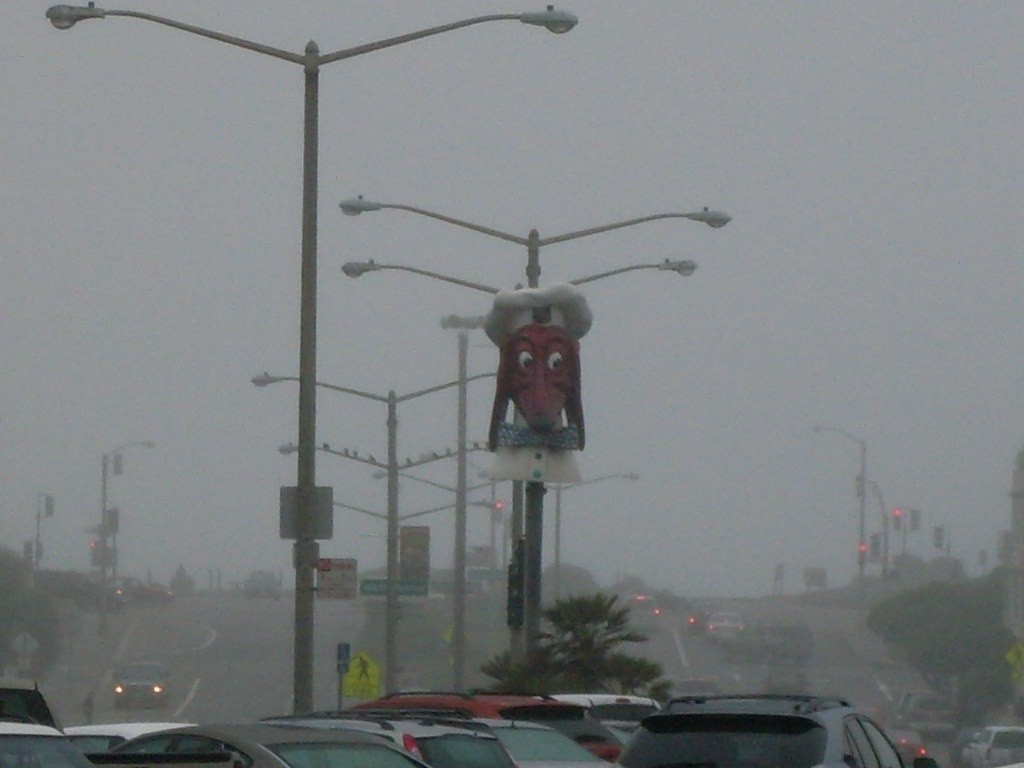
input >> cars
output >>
[113,660,168,710]
[705,612,746,642]
[626,594,660,629]
[0,686,1023,768]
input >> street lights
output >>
[342,259,699,654]
[270,440,644,696]
[252,365,501,697]
[333,189,731,673]
[45,0,580,717]
[812,425,868,632]
[99,437,154,588]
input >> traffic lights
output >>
[493,500,504,523]
[857,544,868,566]
[506,564,524,627]
[893,507,902,531]
